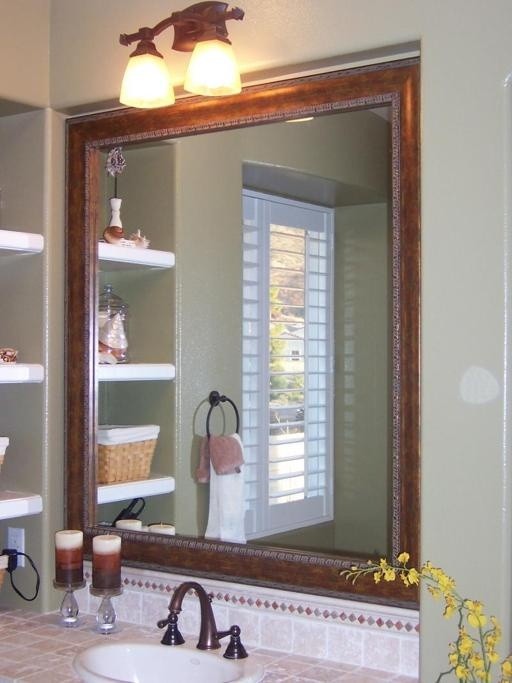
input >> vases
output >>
[109,198,124,228]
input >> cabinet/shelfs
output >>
[95,243,178,508]
[0,230,46,523]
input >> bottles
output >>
[98,283,131,364]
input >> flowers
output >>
[338,555,511,683]
[103,146,128,199]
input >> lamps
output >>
[117,1,248,111]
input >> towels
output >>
[192,429,252,543]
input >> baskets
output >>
[97,425,160,485]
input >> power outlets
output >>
[8,526,26,567]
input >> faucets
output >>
[167,580,220,650]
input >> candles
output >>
[54,531,124,590]
[114,519,175,536]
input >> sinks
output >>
[74,643,241,683]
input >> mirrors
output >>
[58,51,429,614]
